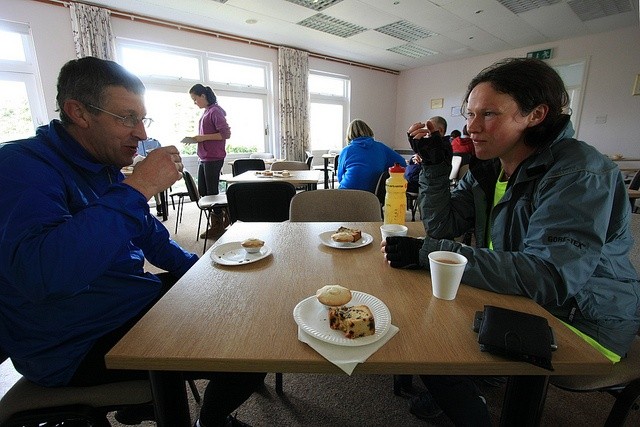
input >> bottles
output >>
[384,163,408,225]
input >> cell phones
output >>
[473,309,559,351]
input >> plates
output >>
[293,291,390,347]
[209,242,272,266]
[317,230,373,249]
[257,175,273,178]
[274,174,294,177]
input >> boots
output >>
[200,212,224,238]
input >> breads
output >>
[334,226,363,242]
[328,305,377,339]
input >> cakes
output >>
[331,233,354,246]
[315,284,352,307]
[242,237,265,253]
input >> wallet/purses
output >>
[477,305,555,372]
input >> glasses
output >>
[87,102,152,128]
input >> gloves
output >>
[408,130,444,164]
[386,235,423,269]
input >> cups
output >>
[379,224,408,240]
[146,148,155,156]
[428,251,468,300]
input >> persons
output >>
[447,130,460,142]
[410,116,447,165]
[337,120,407,190]
[380,78,473,285]
[0,57,267,427]
[462,125,473,138]
[183,83,231,238]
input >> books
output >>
[180,137,199,144]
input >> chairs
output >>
[306,155,315,169]
[183,170,232,254]
[332,155,339,189]
[250,152,274,160]
[170,192,191,234]
[271,161,311,191]
[549,212,640,426]
[627,171,640,211]
[453,153,472,181]
[406,155,462,221]
[232,160,266,175]
[275,189,404,398]
[166,186,176,215]
[374,171,390,221]
[0,280,201,427]
